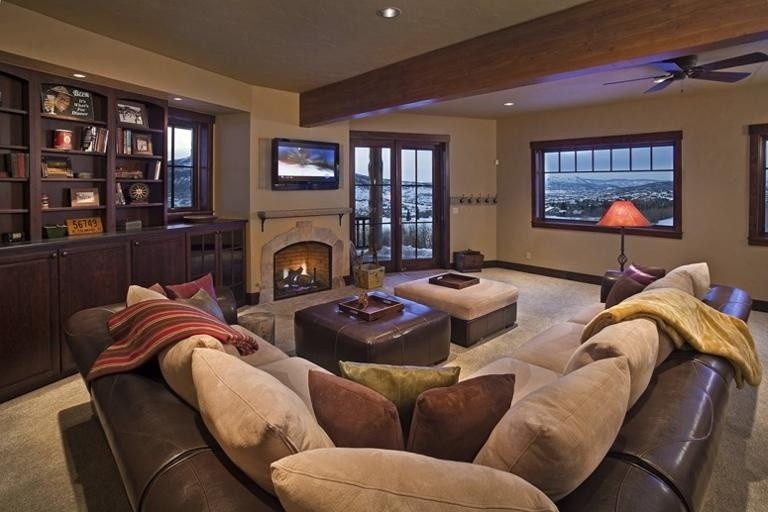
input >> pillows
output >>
[665,262,710,301]
[306,369,403,448]
[339,359,461,438]
[407,373,515,462]
[269,449,560,512]
[642,270,696,309]
[605,263,657,309]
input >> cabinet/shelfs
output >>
[188,220,248,309]
[132,226,188,287]
[0,230,130,403]
[0,50,168,251]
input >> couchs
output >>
[459,285,751,512]
[64,285,336,509]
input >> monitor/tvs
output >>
[271,137,340,191]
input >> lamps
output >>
[597,201,651,271]
[504,103,514,105]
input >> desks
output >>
[394,271,518,346]
[294,289,452,378]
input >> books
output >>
[81,125,131,155]
[146,160,161,180]
[6,153,30,178]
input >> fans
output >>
[603,52,768,94]
